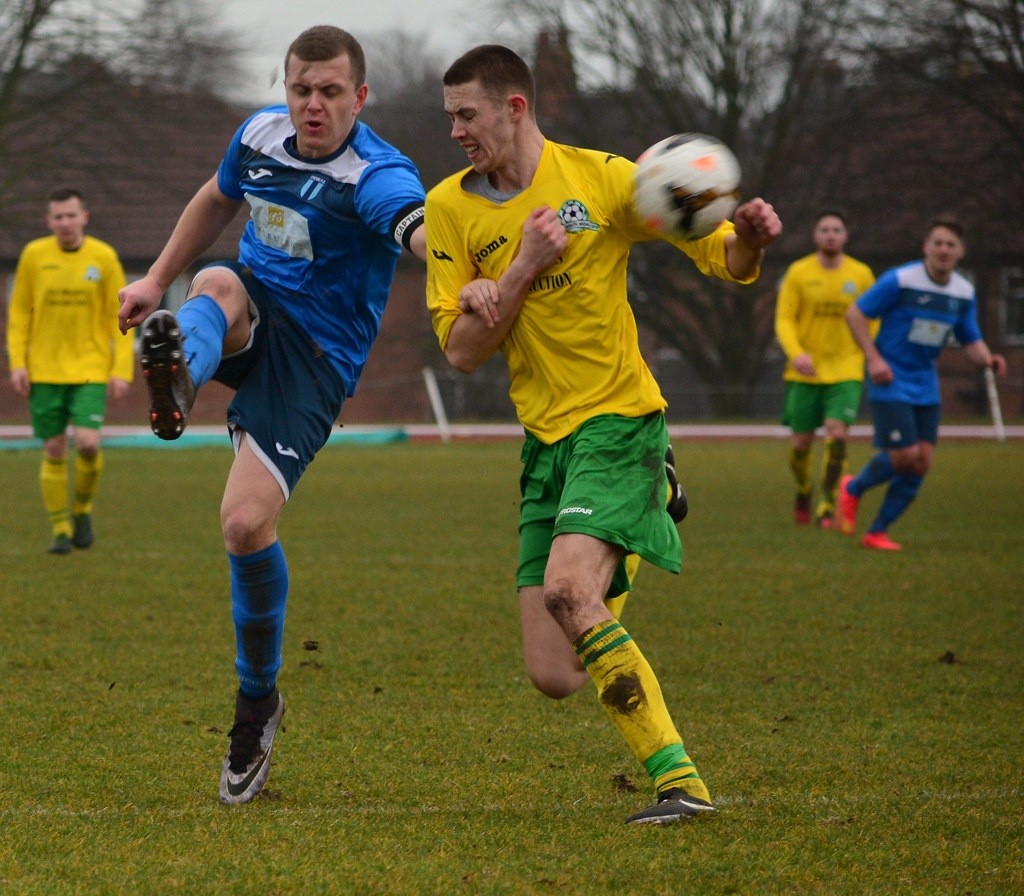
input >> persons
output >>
[834,216,1008,549]
[6,188,132,553]
[422,44,783,823]
[118,23,497,806]
[774,211,883,526]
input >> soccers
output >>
[632,131,743,243]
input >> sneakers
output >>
[819,501,837,527]
[218,685,284,805]
[72,511,92,547]
[50,533,72,553]
[664,442,688,525]
[795,480,811,522]
[139,309,197,440]
[622,788,717,826]
[837,476,860,534]
[863,532,900,550]
